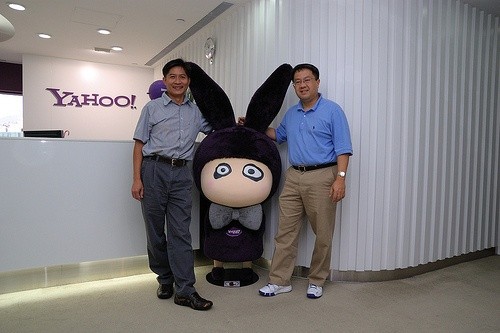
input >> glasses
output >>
[292,77,315,86]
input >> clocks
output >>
[204,37,216,59]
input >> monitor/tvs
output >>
[24,130,64,138]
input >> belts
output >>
[291,162,338,172]
[145,155,188,166]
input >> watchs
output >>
[337,171,346,176]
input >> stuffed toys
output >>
[185,62,294,277]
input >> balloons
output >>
[147,80,167,100]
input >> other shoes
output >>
[174,293,213,311]
[156,284,175,299]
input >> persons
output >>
[131,59,238,311]
[238,64,353,299]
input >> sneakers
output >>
[259,283,293,296]
[307,284,323,299]
